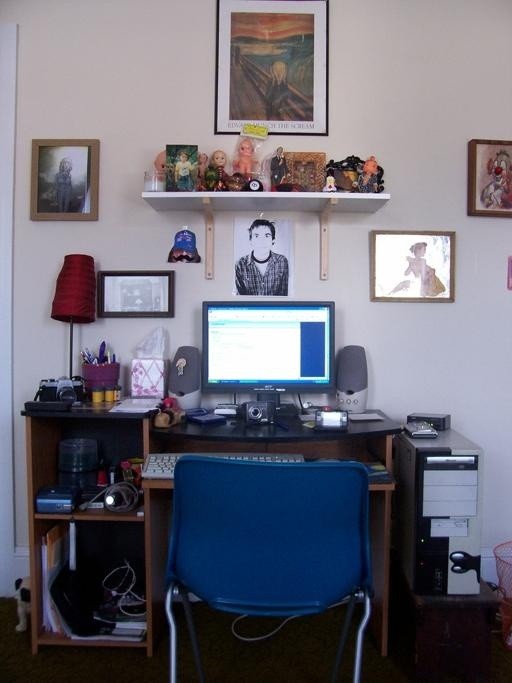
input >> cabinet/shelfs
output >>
[19,396,406,661]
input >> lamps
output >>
[51,253,94,380]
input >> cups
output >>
[80,362,120,400]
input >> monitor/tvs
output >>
[201,300,335,399]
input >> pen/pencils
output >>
[80,341,115,366]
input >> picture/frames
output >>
[29,139,98,220]
[467,138,511,217]
[96,271,174,318]
[213,1,328,136]
[369,229,455,304]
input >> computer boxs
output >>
[397,429,481,597]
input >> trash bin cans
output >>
[493,541,512,652]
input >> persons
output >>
[265,62,289,122]
[405,242,445,297]
[153,140,385,193]
[55,157,72,212]
[235,220,288,296]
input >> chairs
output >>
[165,456,373,683]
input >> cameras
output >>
[243,401,278,423]
[316,411,349,430]
[39,379,84,404]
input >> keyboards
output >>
[141,452,306,479]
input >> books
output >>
[116,617,147,630]
[70,628,146,643]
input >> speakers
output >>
[169,346,202,410]
[335,346,368,411]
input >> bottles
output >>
[142,169,167,193]
[91,385,121,403]
